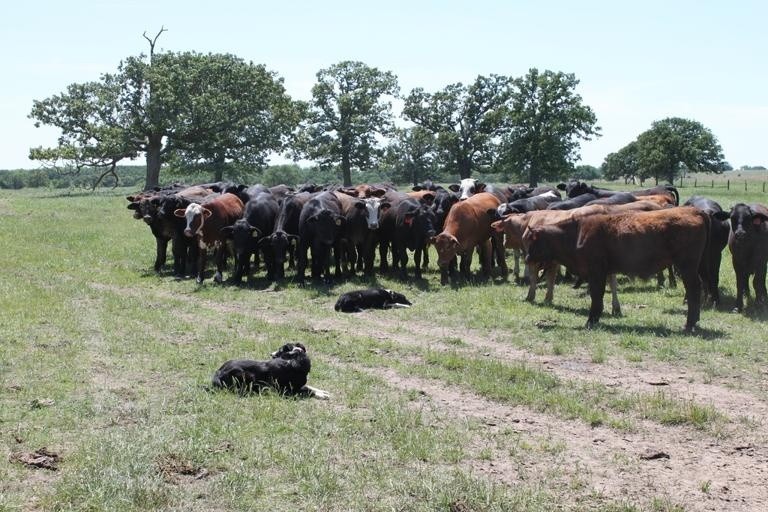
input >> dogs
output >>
[210,343,331,401]
[333,288,415,315]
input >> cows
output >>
[126,176,502,287]
[715,197,768,316]
[505,177,728,330]
